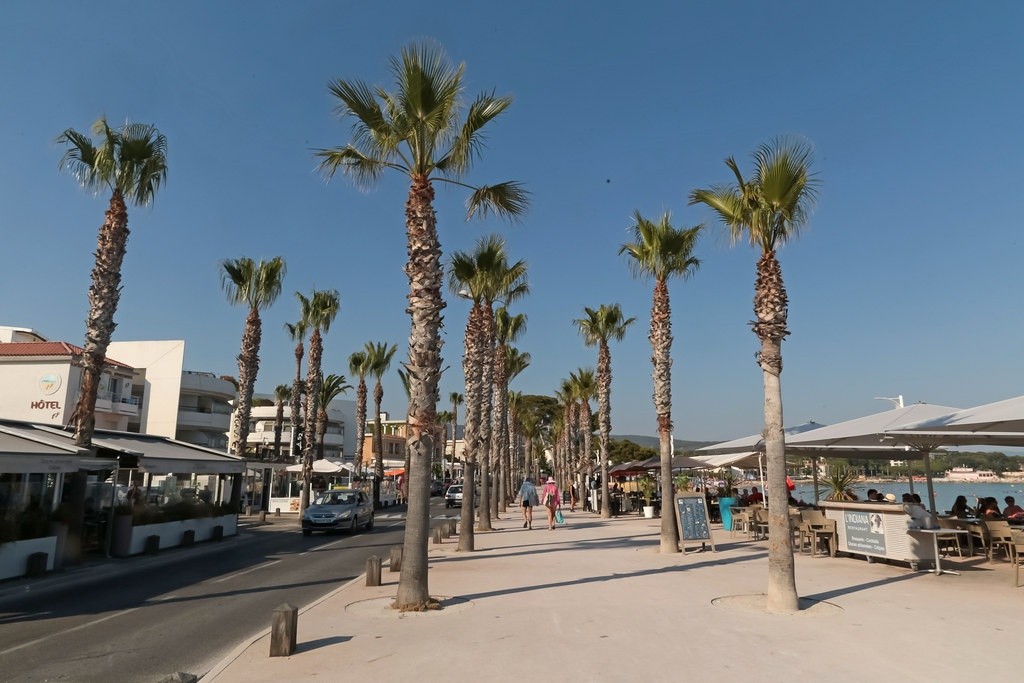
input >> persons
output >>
[865,489,878,502]
[329,495,337,504]
[311,476,407,505]
[610,483,763,515]
[202,485,212,503]
[952,496,1024,519]
[444,478,458,494]
[518,477,537,530]
[347,495,353,503]
[569,478,576,512]
[127,480,138,508]
[785,490,798,507]
[541,476,561,531]
[883,494,897,503]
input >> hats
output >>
[546,476,555,483]
[882,493,896,502]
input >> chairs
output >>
[788,506,838,558]
[705,496,721,523]
[728,502,770,542]
[937,510,1024,586]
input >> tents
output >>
[284,459,406,475]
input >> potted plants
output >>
[113,502,134,557]
[48,504,74,567]
[637,476,657,519]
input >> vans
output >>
[445,485,476,508]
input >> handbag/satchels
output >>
[529,485,537,506]
[554,509,563,523]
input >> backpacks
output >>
[542,492,553,507]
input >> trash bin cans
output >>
[27,553,48,579]
[147,536,160,553]
[184,530,195,547]
[214,525,223,541]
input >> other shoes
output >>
[549,524,555,530]
[529,526,531,530]
[524,521,527,528]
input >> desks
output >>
[920,529,969,558]
[947,517,983,523]
[615,494,660,512]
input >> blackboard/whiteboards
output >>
[674,492,714,542]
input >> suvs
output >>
[302,489,375,535]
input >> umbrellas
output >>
[572,395,1024,508]
